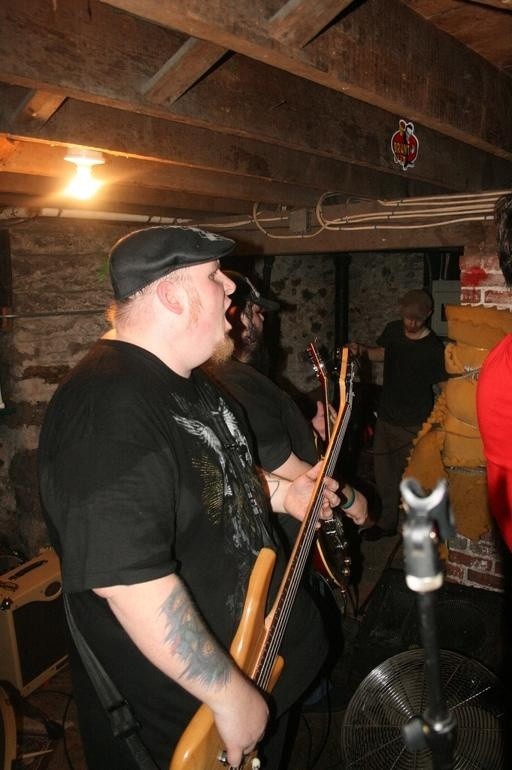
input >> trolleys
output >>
[339,468,508,770]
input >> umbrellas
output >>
[361,526,398,541]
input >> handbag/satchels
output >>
[341,483,355,510]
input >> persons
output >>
[37,225,340,770]
[342,290,448,537]
[476,193,512,557]
[202,271,368,547]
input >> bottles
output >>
[399,289,432,321]
[107,223,237,300]
[222,270,280,311]
